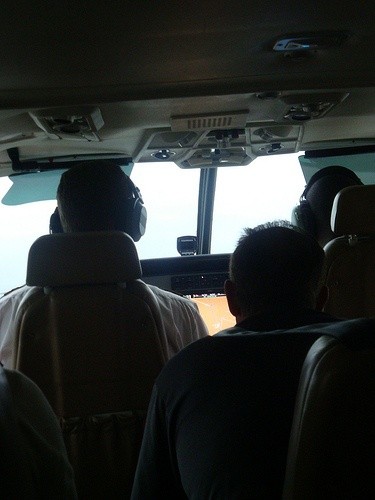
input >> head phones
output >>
[47,164,147,242]
[293,165,363,229]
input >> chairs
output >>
[312,182,375,321]
[12,229,170,500]
[280,315,375,500]
[0,366,78,500]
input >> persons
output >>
[1,364,80,500]
[0,158,209,374]
[292,163,366,248]
[132,219,348,500]
